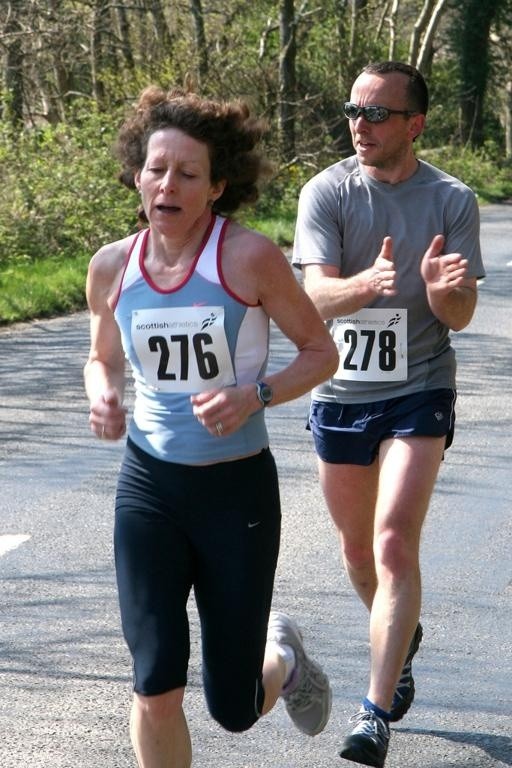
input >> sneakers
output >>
[267,609,333,735]
[340,703,391,767]
[390,621,423,722]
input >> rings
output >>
[100,425,105,439]
[215,421,223,433]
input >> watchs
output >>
[254,380,274,407]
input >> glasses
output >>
[342,100,416,125]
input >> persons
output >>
[84,84,339,768]
[291,60,486,768]
